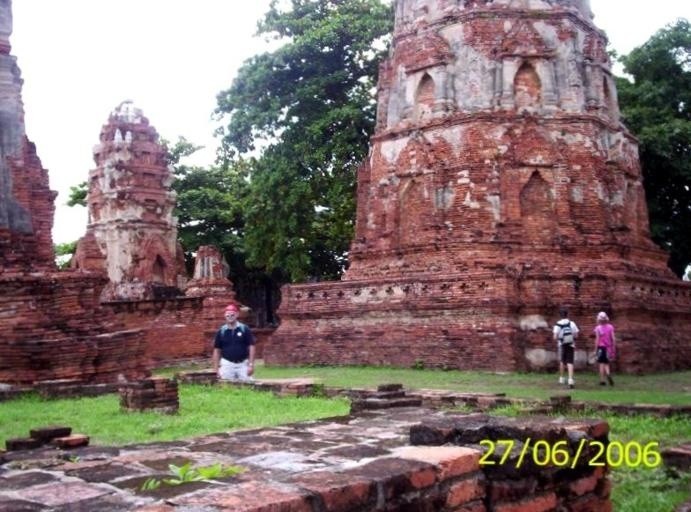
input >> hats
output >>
[597,311,610,320]
[225,304,238,311]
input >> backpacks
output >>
[555,322,574,346]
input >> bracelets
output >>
[248,362,255,367]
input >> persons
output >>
[211,304,259,380]
[554,309,581,390]
[593,311,617,387]
